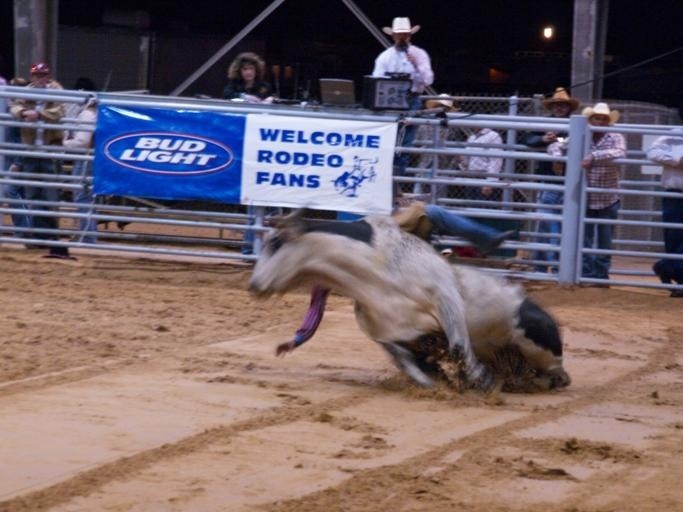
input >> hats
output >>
[542,86,579,112]
[228,52,266,80]
[425,93,461,112]
[581,102,620,125]
[383,17,422,35]
[30,62,49,75]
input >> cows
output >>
[245,204,572,397]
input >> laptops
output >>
[319,76,362,108]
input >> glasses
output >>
[592,115,610,123]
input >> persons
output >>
[0,63,98,245]
[647,126,683,297]
[413,93,504,227]
[525,86,626,288]
[372,17,435,198]
[272,200,518,357]
[223,53,271,100]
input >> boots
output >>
[424,202,519,257]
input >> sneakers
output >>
[651,263,683,298]
[580,284,610,288]
